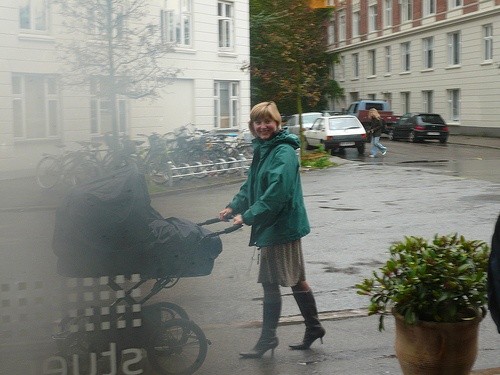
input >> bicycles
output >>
[36,124,254,190]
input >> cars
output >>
[284,112,323,139]
[301,115,367,156]
[389,112,448,144]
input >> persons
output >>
[368,108,388,153]
[218,101,325,359]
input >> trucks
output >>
[342,99,402,143]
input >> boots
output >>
[289,288,326,349]
[239,300,282,359]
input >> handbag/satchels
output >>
[52,172,223,277]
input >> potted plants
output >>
[353,233,491,374]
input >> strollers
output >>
[44,167,245,375]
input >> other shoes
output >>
[381,147,387,156]
[369,154,377,158]
